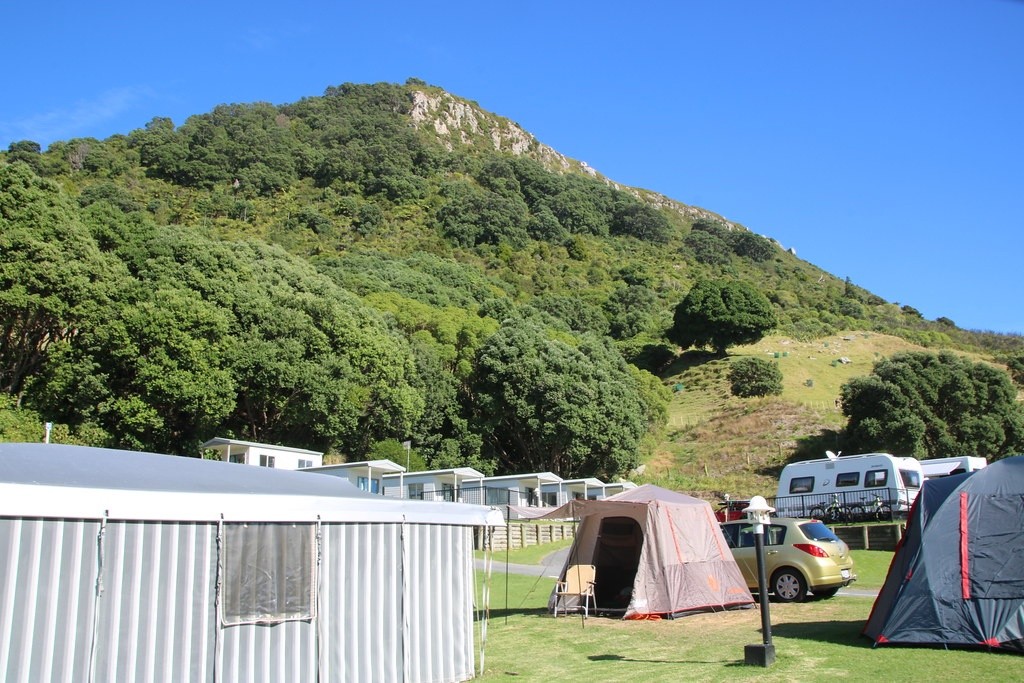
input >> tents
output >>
[503,480,759,626]
[2,437,505,683]
[859,453,1023,653]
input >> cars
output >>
[720,518,858,602]
[715,503,748,521]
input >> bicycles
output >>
[848,493,894,524]
[809,493,853,525]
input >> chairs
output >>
[554,563,598,621]
